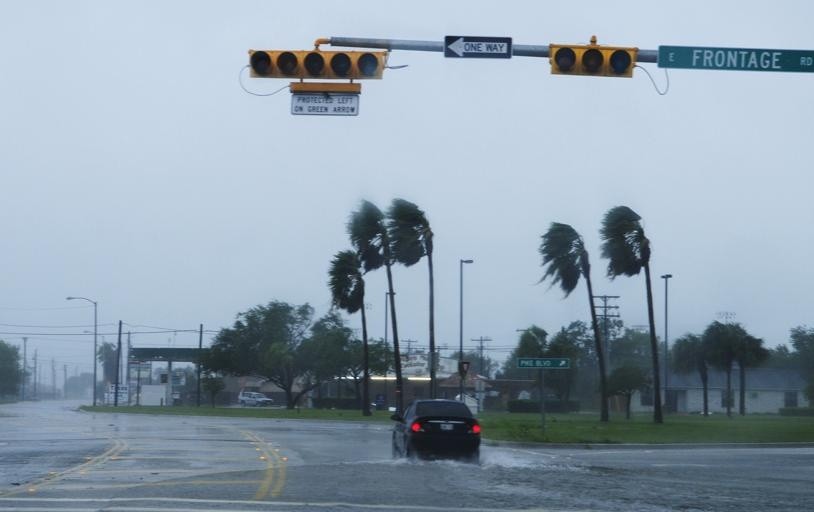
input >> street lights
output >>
[660,273,673,413]
[383,291,397,410]
[82,328,108,407]
[65,294,98,406]
[457,258,474,403]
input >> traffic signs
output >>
[656,43,813,74]
[441,34,514,58]
[290,93,361,116]
[517,356,570,370]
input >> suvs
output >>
[237,391,275,408]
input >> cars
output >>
[389,397,481,467]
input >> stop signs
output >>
[394,388,401,395]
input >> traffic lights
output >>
[545,43,640,80]
[246,46,388,80]
